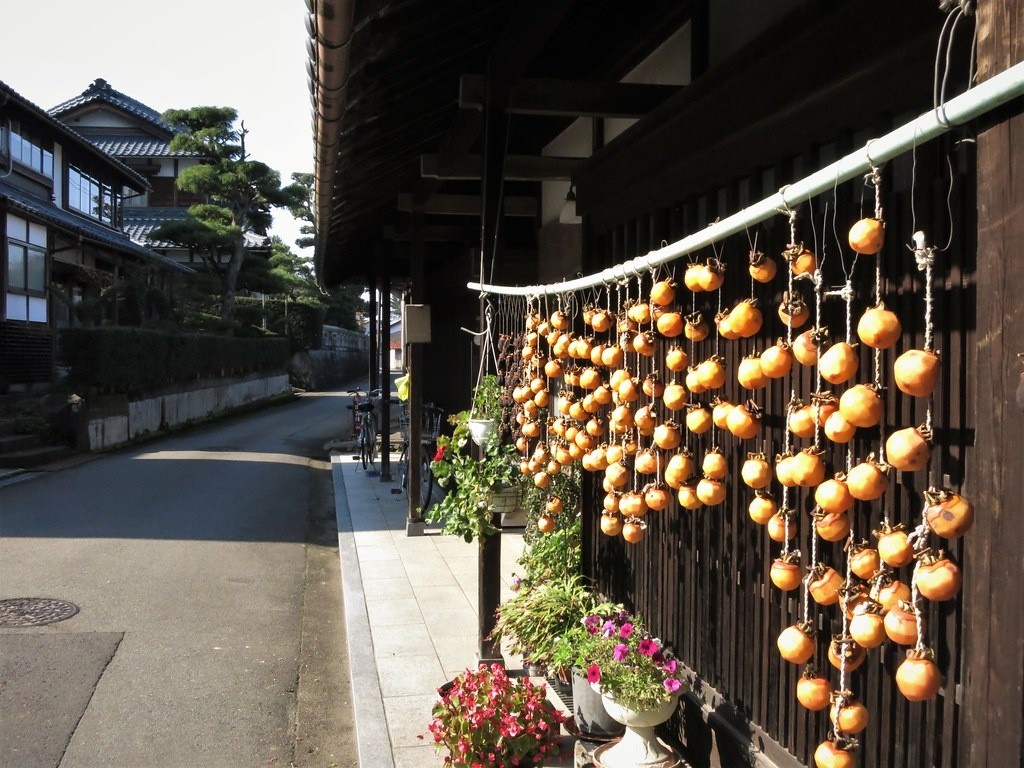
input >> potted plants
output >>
[547,603,627,739]
[455,373,509,447]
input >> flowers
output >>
[581,610,683,712]
[425,433,519,543]
[416,661,575,767]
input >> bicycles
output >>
[387,398,445,518]
[346,386,385,475]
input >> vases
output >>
[490,486,519,513]
[590,681,679,768]
